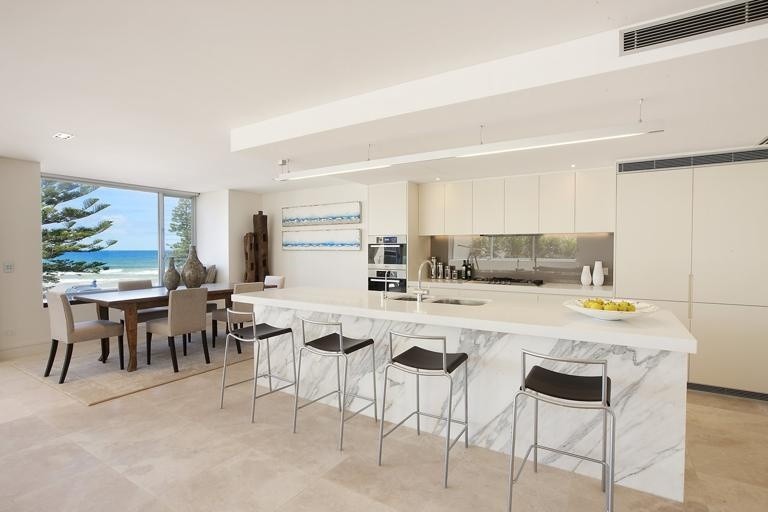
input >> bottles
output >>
[444,265,450,279]
[452,269,458,279]
[467,264,471,280]
[462,260,467,279]
[436,262,444,279]
[430,257,439,279]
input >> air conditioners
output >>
[620,0,768,55]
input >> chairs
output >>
[262,275,285,290]
[176,303,219,342]
[220,307,298,423]
[211,282,267,352]
[377,329,468,487]
[294,315,377,450]
[119,280,169,327]
[44,291,125,384]
[508,347,615,512]
[145,288,211,372]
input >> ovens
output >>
[367,235,407,293]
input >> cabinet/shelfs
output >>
[417,182,445,236]
[537,171,575,234]
[615,145,768,400]
[369,182,431,281]
[446,181,473,235]
[473,178,504,235]
[575,165,617,234]
[503,176,538,236]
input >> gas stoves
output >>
[465,277,543,292]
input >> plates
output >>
[561,298,659,321]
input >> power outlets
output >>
[4,263,14,272]
